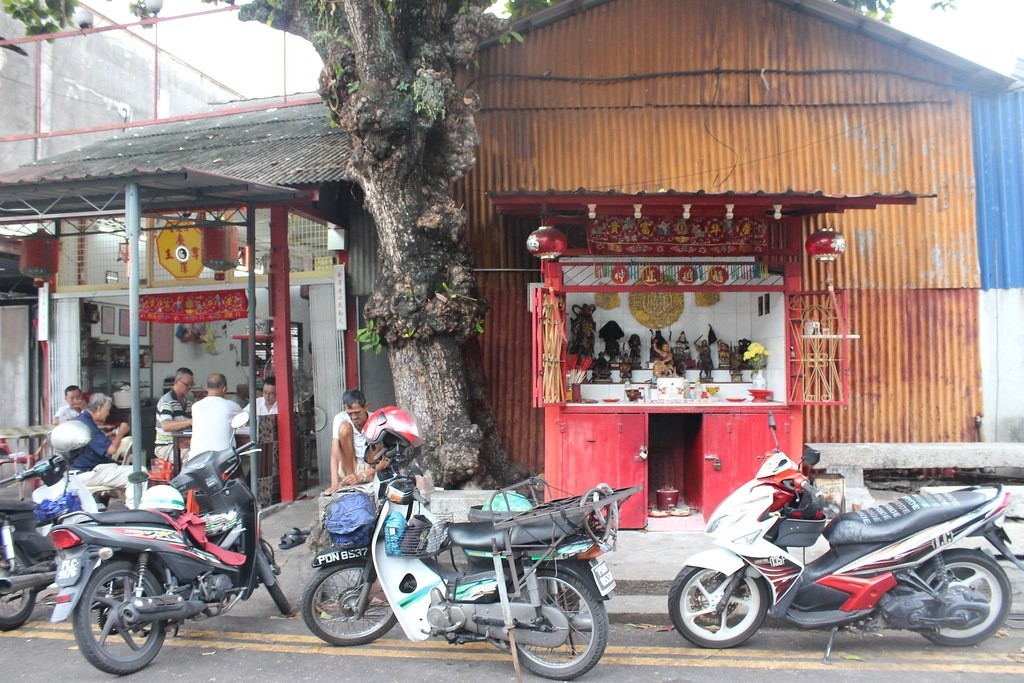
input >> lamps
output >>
[682,204,692,217]
[76,9,93,28]
[587,204,597,218]
[632,204,643,218]
[773,204,783,218]
[725,203,736,219]
[146,0,163,13]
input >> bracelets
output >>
[361,472,369,482]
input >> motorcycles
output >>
[0,412,296,677]
[302,439,644,679]
[667,410,1024,667]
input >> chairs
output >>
[109,436,133,466]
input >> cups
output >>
[571,383,580,403]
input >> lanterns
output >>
[201,224,239,280]
[805,229,846,262]
[525,226,567,260]
[18,228,58,287]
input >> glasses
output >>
[178,378,197,388]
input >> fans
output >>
[263,344,314,411]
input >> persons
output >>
[154,367,196,463]
[325,390,390,495]
[188,372,246,481]
[242,376,278,416]
[66,392,148,509]
[572,302,744,383]
[52,385,87,426]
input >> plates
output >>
[725,397,746,402]
[601,398,620,402]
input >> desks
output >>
[0,423,61,502]
[172,426,251,478]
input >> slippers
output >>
[278,535,306,549]
[280,527,311,541]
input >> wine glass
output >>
[706,384,720,401]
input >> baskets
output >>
[383,513,454,559]
[322,493,379,551]
[197,505,239,537]
[33,489,82,530]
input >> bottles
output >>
[689,382,702,399]
[623,382,631,401]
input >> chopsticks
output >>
[570,369,587,383]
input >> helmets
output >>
[50,419,92,453]
[784,489,825,521]
[138,485,186,516]
[362,405,415,448]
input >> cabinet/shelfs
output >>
[699,413,796,524]
[559,414,650,532]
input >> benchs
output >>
[318,489,517,528]
[803,442,1024,560]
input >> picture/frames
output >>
[100,306,175,363]
